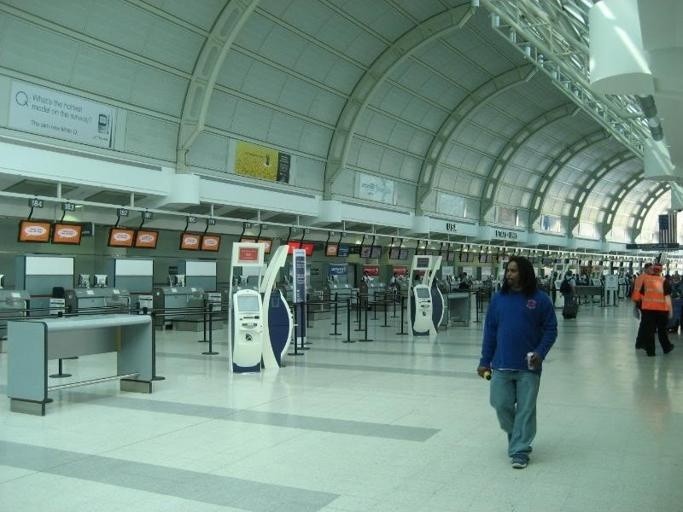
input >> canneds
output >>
[527,352,534,369]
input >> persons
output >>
[390,272,400,286]
[478,256,558,468]
[457,272,469,285]
[362,271,372,282]
[538,263,683,356]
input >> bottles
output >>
[483,370,491,380]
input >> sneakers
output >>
[512,454,528,468]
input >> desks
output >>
[576,285,604,306]
[438,291,482,327]
[6,312,166,416]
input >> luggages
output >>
[563,303,578,319]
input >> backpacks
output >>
[560,275,573,292]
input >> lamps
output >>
[589,0,683,211]
